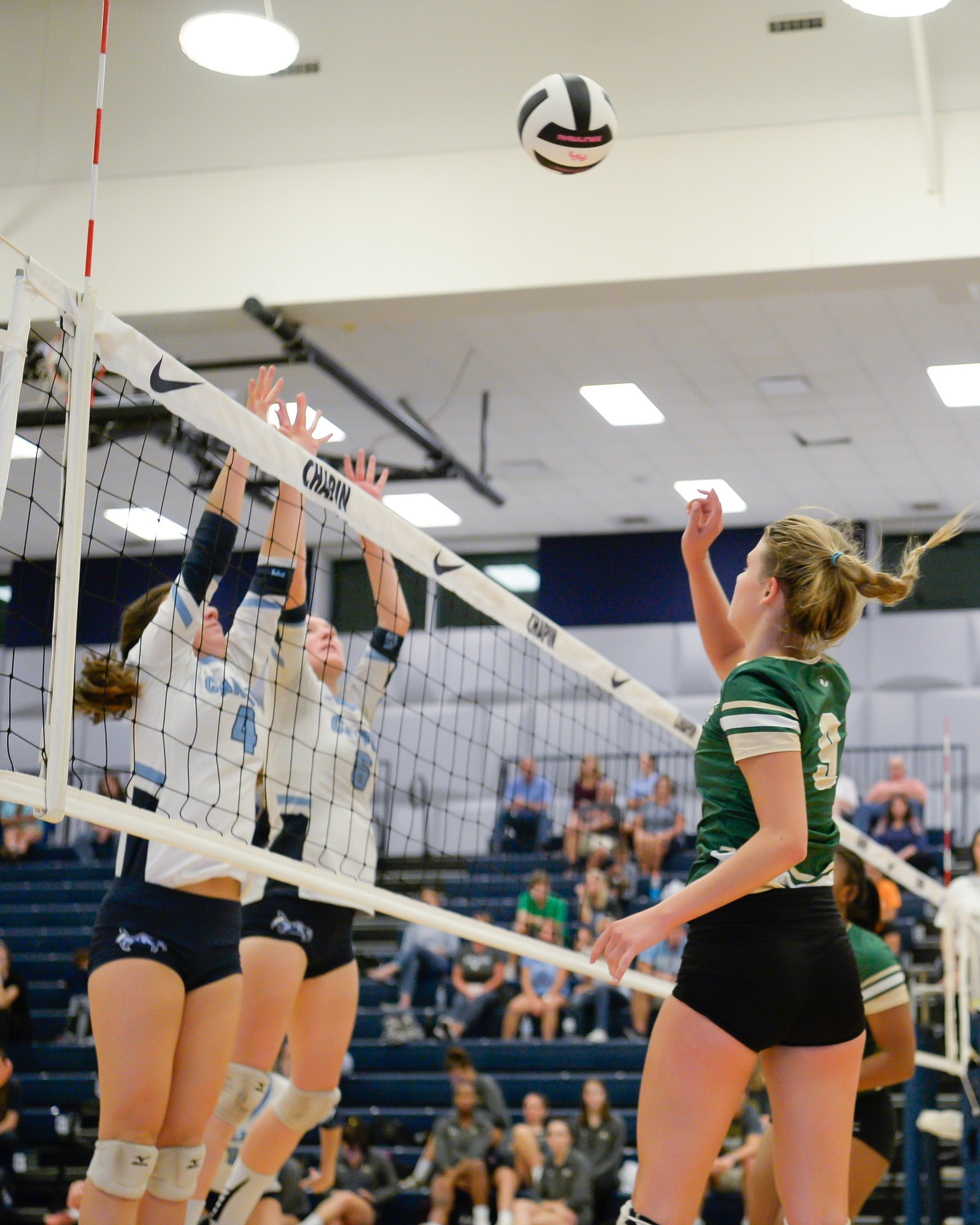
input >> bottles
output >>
[519,1015,534,1042]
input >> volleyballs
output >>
[517,73,617,174]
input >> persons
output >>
[0,365,980,1225]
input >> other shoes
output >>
[434,1019,455,1043]
[585,1028,609,1043]
[473,1205,490,1225]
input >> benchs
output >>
[0,827,944,1211]
